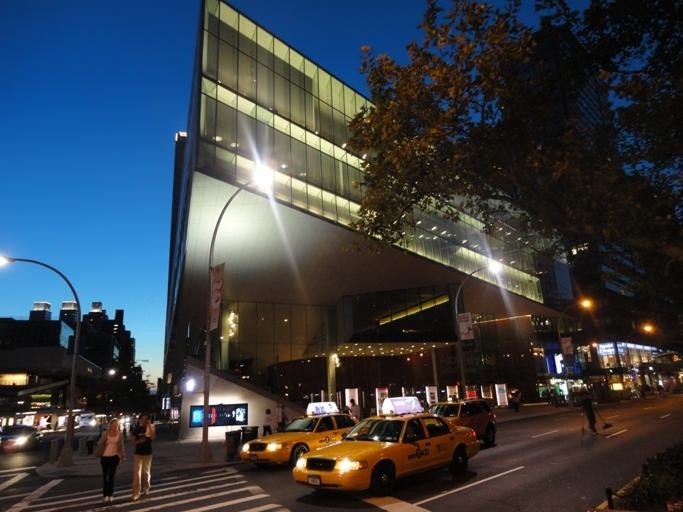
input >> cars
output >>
[0,424,44,454]
[238,397,497,497]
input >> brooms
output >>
[593,406,612,430]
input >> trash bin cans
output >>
[241,426,259,445]
[225,430,241,461]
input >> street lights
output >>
[555,298,593,406]
[198,165,272,462]
[451,260,506,402]
[0,254,82,469]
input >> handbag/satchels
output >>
[94,431,108,458]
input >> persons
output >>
[579,391,600,439]
[549,389,557,408]
[543,390,551,408]
[349,398,358,419]
[261,408,272,437]
[511,392,520,412]
[95,417,127,505]
[129,412,157,502]
[275,405,289,432]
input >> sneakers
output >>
[130,490,148,502]
[104,495,113,502]
